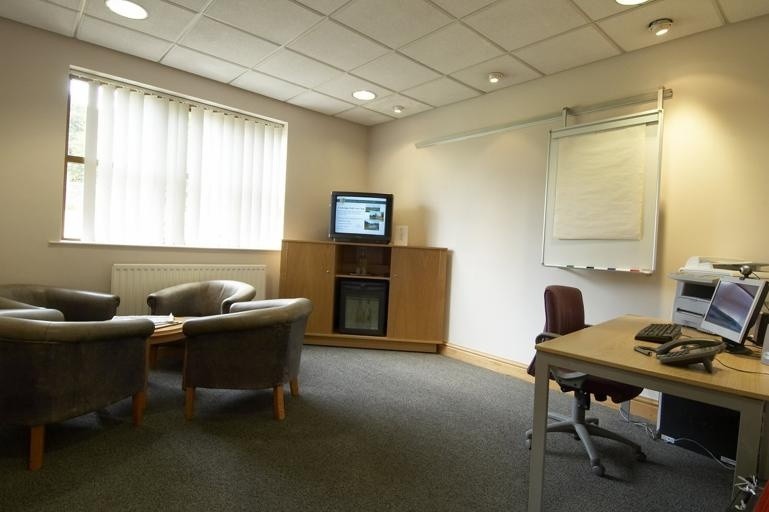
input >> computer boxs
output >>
[656,391,740,467]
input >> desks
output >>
[527,315,769,512]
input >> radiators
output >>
[111,264,266,315]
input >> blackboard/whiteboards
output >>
[541,108,664,278]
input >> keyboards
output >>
[634,322,681,341]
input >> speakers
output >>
[753,312,769,346]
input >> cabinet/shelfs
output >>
[279,240,447,353]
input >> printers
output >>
[667,255,769,330]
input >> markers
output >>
[565,264,629,272]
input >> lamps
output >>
[648,19,672,36]
[394,106,403,113]
[488,73,503,83]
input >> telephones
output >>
[656,339,727,374]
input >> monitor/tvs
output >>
[329,191,394,245]
[700,276,769,355]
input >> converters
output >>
[661,432,679,444]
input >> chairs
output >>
[0,285,120,320]
[183,297,313,420]
[0,308,155,471]
[525,286,650,475]
[147,280,257,316]
